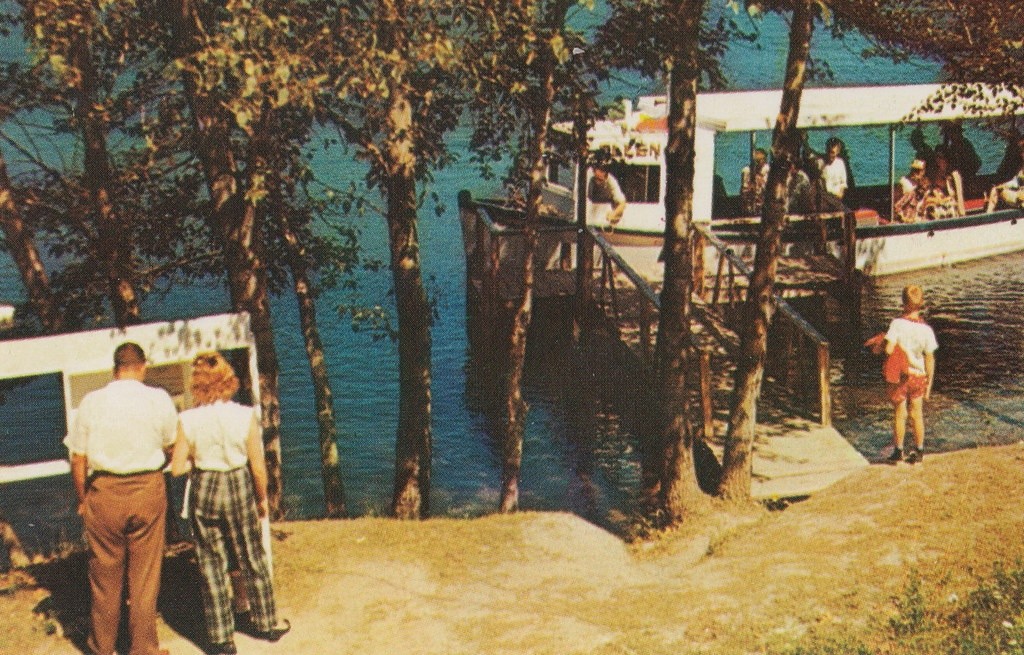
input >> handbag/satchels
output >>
[181,477,192,519]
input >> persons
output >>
[816,137,848,201]
[986,148,1024,213]
[894,148,965,224]
[62,343,177,655]
[883,284,939,463]
[172,352,291,655]
[587,164,626,225]
[741,148,771,216]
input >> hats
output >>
[910,160,926,169]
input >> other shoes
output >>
[266,619,292,642]
[213,642,237,655]
[903,456,922,467]
[885,453,903,467]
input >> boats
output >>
[456,82,1023,307]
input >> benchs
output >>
[854,207,880,226]
[960,192,985,214]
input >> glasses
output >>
[194,356,218,368]
